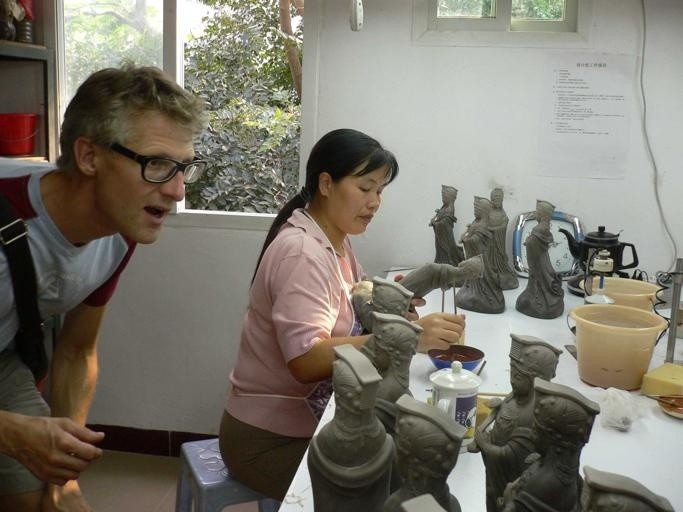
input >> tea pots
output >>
[558,225,639,272]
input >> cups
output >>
[429,358,483,449]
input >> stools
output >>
[176,438,278,512]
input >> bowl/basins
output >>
[428,343,485,371]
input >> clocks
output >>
[512,212,586,280]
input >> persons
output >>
[350,250,486,335]
[464,330,564,512]
[491,374,602,512]
[305,341,396,511]
[365,309,425,409]
[429,184,464,268]
[370,274,417,329]
[514,198,566,320]
[457,194,506,315]
[376,391,469,510]
[0,53,212,512]
[216,128,468,503]
[482,186,522,290]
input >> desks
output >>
[278,264,683,512]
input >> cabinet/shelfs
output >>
[0,0,60,183]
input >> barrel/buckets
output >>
[0,111,39,156]
[565,303,672,390]
[578,275,664,313]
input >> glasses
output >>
[110,142,208,185]
